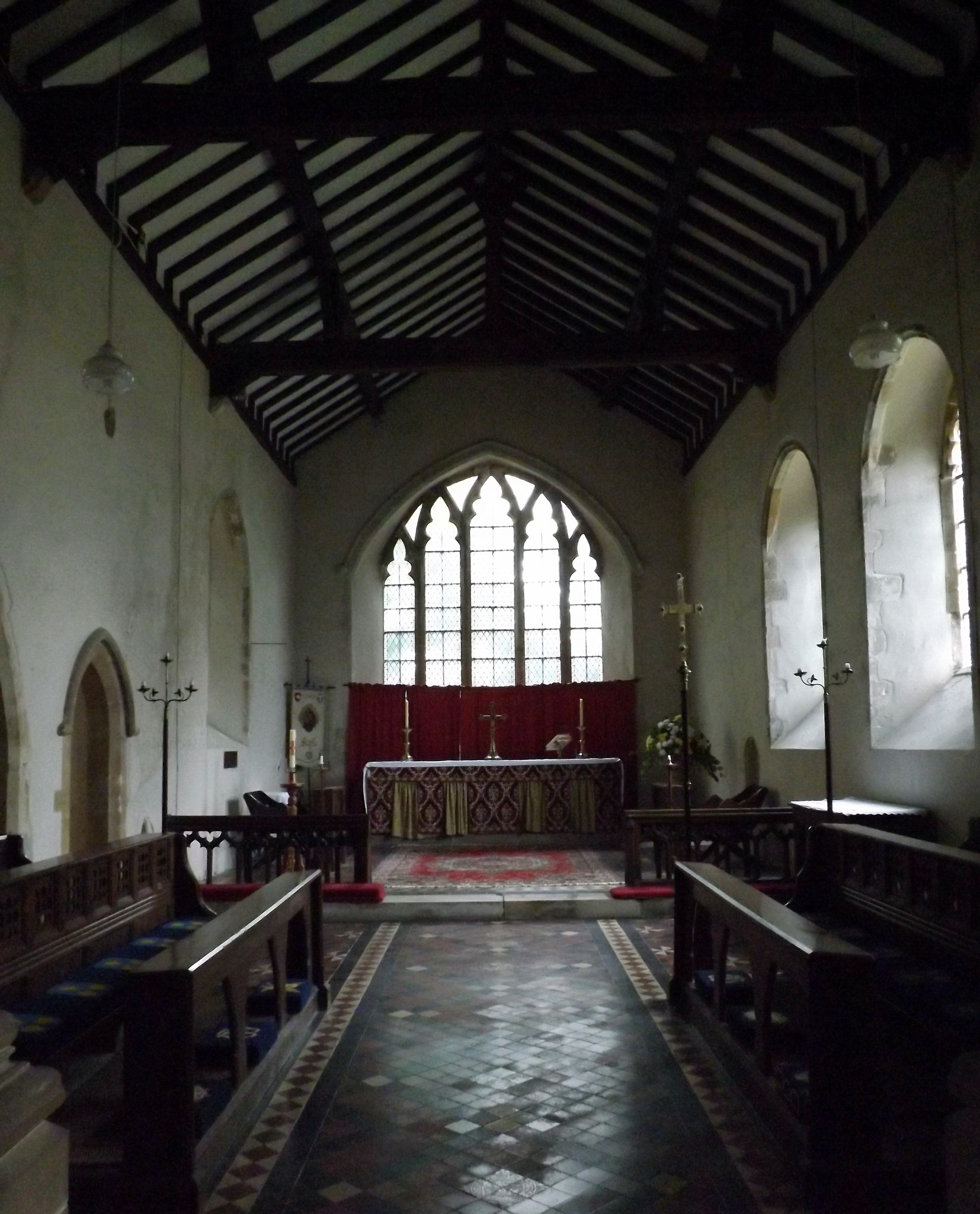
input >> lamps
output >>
[83,1,138,435]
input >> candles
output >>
[403,690,410,728]
[845,662,850,670]
[822,638,826,643]
[290,729,297,768]
[579,698,585,727]
[797,667,802,672]
[319,755,324,764]
